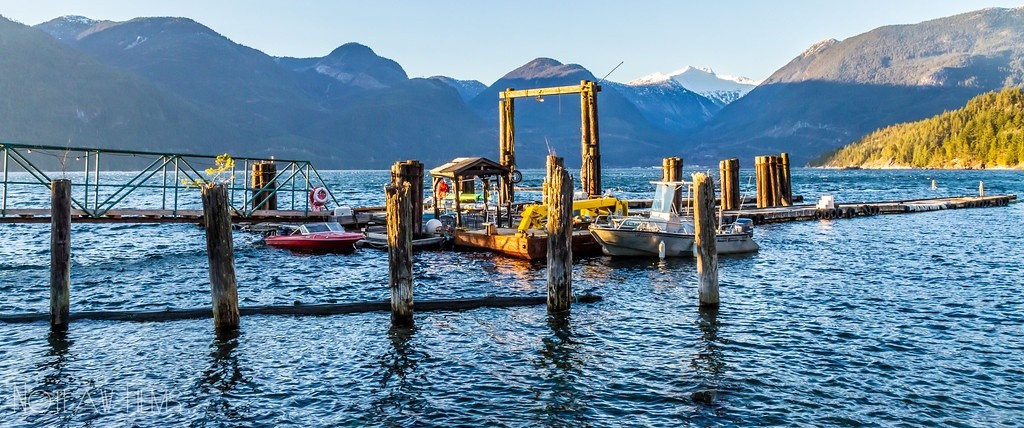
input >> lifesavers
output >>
[314,187,329,203]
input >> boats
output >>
[264,220,366,252]
[587,181,761,257]
